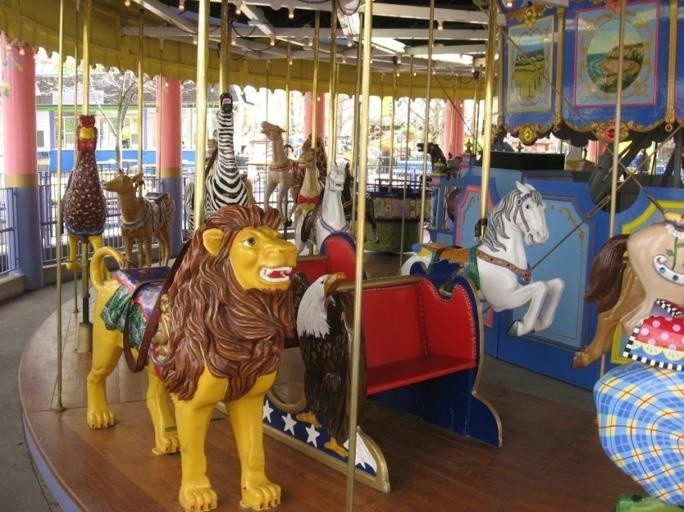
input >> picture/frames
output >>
[573,0,660,108]
[507,14,554,114]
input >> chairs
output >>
[214,231,504,494]
